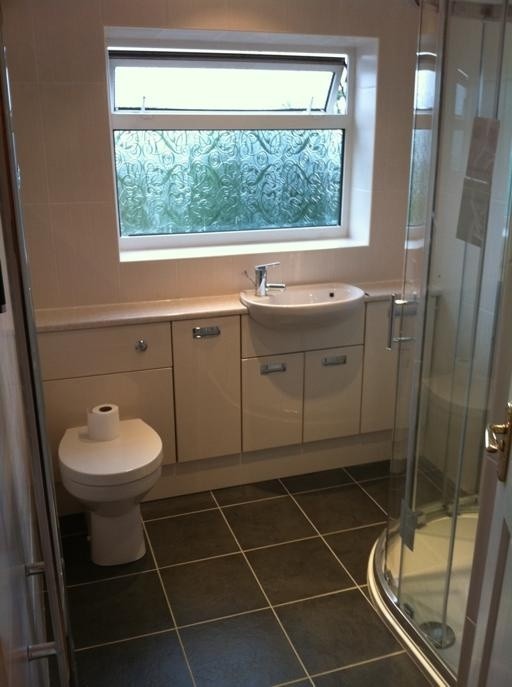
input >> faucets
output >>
[244,259,286,296]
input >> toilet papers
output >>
[86,402,122,440]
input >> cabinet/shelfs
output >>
[170,317,241,496]
[37,323,172,383]
[243,346,363,486]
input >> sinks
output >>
[241,283,366,328]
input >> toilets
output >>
[56,418,163,567]
